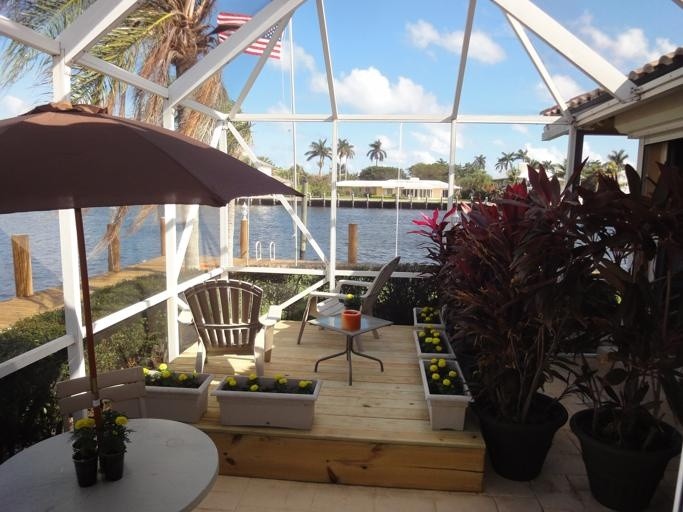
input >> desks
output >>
[0,416,221,512]
[307,314,395,386]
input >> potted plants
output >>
[440,154,633,482]
[500,149,683,512]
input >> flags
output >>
[216,12,281,59]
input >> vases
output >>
[418,359,476,432]
[413,307,446,330]
[210,374,323,432]
[69,439,128,487]
[413,329,456,360]
[110,369,214,422]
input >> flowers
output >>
[344,294,361,308]
[86,418,96,443]
[143,363,200,388]
[418,324,443,352]
[430,357,457,389]
[71,419,95,458]
[419,306,439,323]
[100,409,136,453]
[227,373,313,394]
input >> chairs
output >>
[56,366,148,429]
[178,278,282,377]
[298,255,401,353]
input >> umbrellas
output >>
[0,100,305,401]
